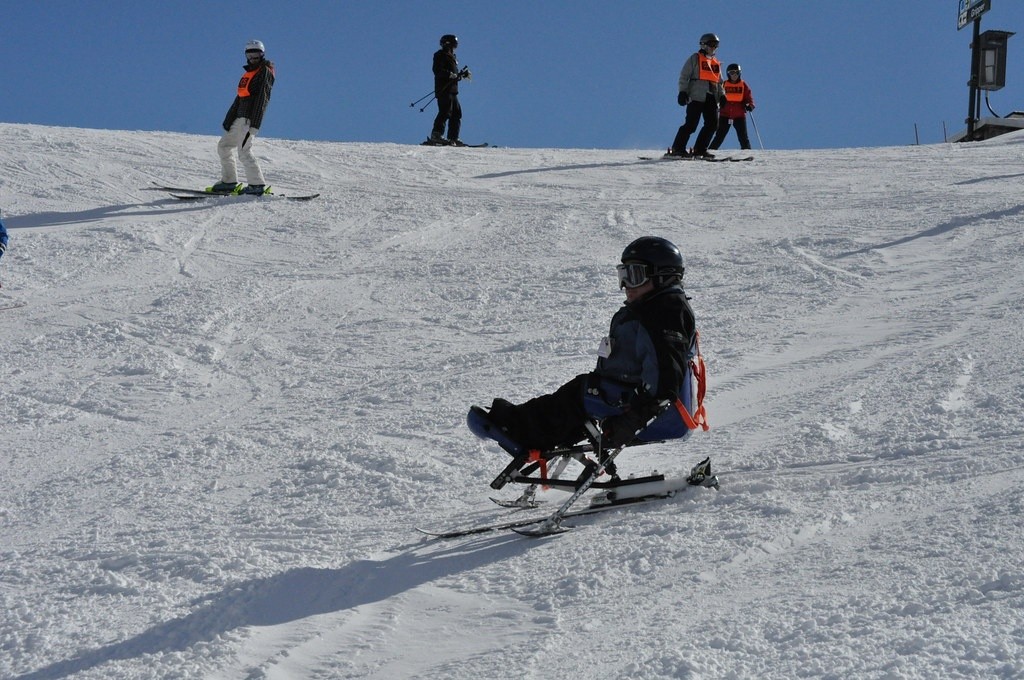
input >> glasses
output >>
[246,52,259,59]
[728,70,741,75]
[704,42,717,49]
[616,263,650,290]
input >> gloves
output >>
[746,105,753,112]
[717,95,726,107]
[249,127,259,136]
[677,91,689,106]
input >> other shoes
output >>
[467,398,535,462]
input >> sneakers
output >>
[694,149,715,158]
[431,134,449,143]
[238,185,264,195]
[672,147,691,158]
[211,182,237,192]
[447,138,464,147]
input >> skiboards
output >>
[151,179,321,202]
[413,495,677,538]
[638,154,756,162]
[418,142,499,148]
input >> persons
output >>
[205,39,276,194]
[708,63,755,150]
[432,34,463,146]
[672,33,727,158]
[467,237,695,451]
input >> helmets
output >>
[621,236,685,283]
[439,34,458,49]
[700,32,720,44]
[726,63,742,75]
[244,40,265,52]
